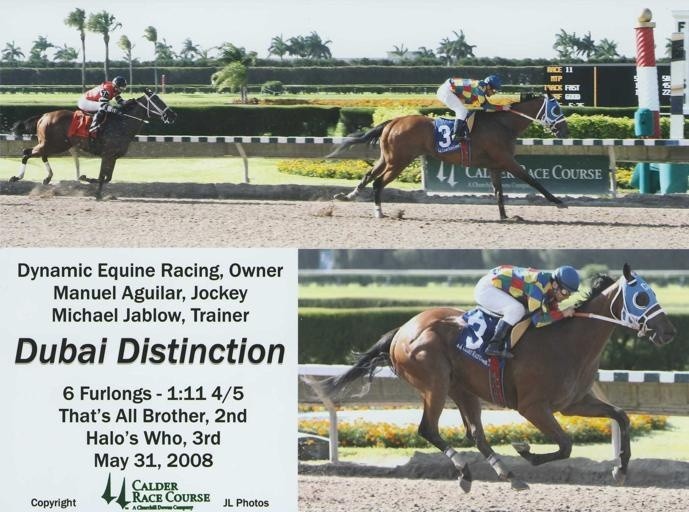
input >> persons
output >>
[77,73,131,134]
[435,72,510,142]
[470,261,581,360]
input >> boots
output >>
[90,110,106,131]
[484,319,514,359]
[452,119,466,141]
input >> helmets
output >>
[484,75,501,91]
[551,265,580,292]
[113,77,127,92]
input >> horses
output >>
[299,262,678,495]
[10,86,178,201]
[326,85,569,220]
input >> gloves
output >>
[111,106,122,116]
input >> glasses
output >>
[556,268,570,297]
[487,77,496,93]
